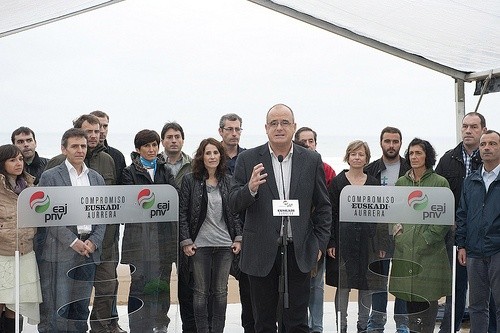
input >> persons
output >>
[227,103,331,333]
[0,110,500,333]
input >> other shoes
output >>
[155,326,168,333]
[111,322,127,333]
[89,323,114,333]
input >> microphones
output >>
[278,155,286,200]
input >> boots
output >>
[0,311,24,333]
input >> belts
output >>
[78,233,90,241]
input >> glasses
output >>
[221,127,244,133]
[266,121,294,128]
